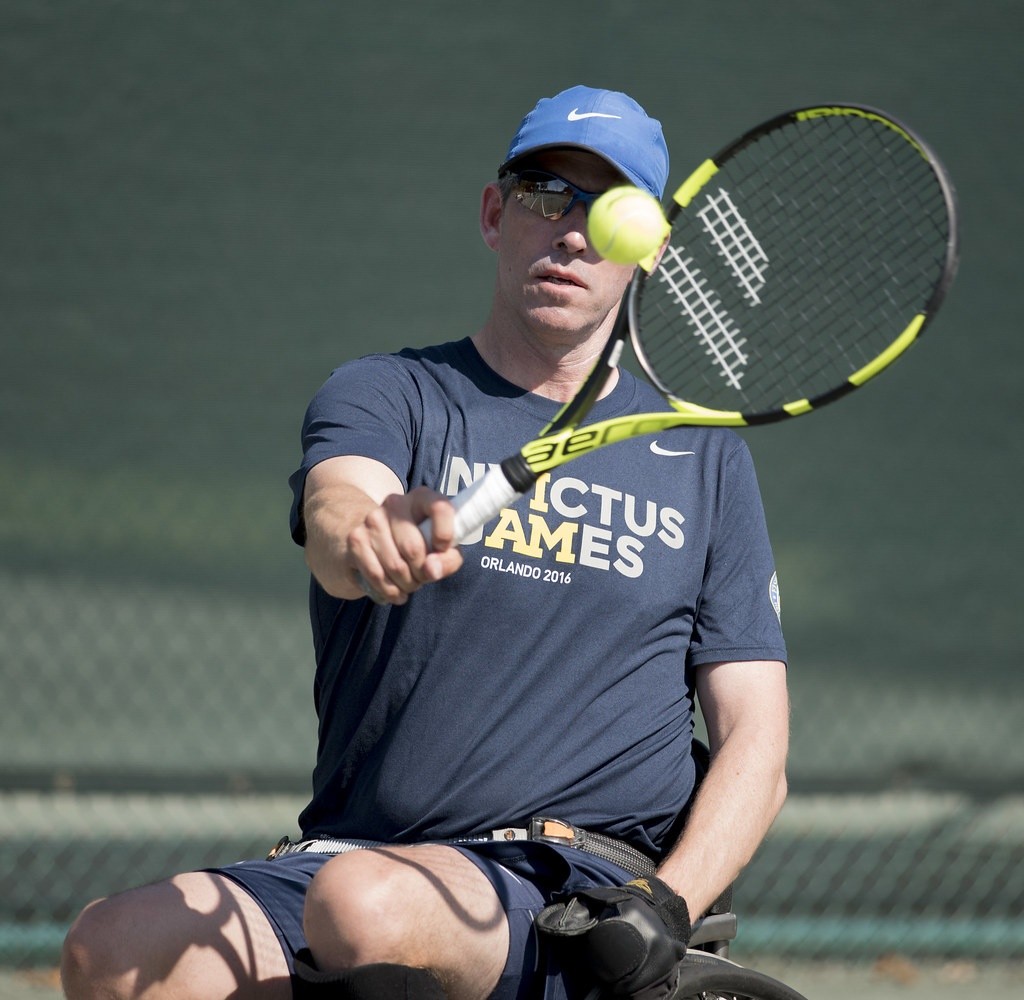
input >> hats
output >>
[502,85,670,202]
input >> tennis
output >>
[588,188,667,267]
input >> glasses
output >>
[498,162,606,221]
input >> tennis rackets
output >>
[413,102,959,554]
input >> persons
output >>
[63,85,791,999]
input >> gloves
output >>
[536,874,691,1000]
